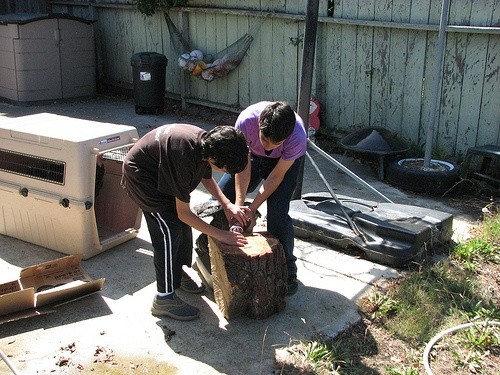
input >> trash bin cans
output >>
[131,51,169,116]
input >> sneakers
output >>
[286,272,299,294]
[152,292,201,321]
[195,234,209,255]
[176,270,207,293]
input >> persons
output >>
[209,101,306,296]
[120,124,251,320]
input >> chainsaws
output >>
[194,199,261,236]
